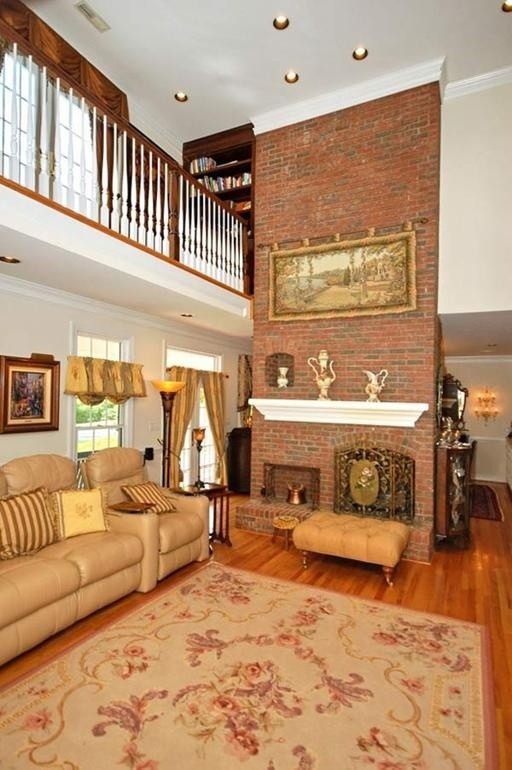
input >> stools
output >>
[271,511,410,589]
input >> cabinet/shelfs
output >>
[182,123,255,240]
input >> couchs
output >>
[0,456,144,667]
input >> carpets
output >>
[0,560,501,768]
[471,484,505,523]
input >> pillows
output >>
[0,486,111,559]
[120,481,177,515]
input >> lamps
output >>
[150,380,207,488]
[473,384,500,426]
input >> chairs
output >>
[84,446,212,594]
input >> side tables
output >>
[179,483,234,555]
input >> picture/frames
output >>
[267,232,416,321]
[0,356,60,433]
